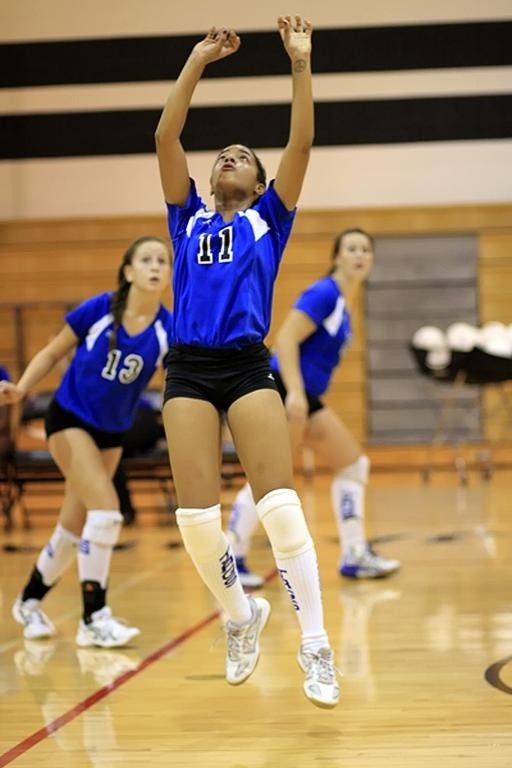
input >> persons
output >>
[1,236,173,647]
[222,228,402,589]
[153,13,343,708]
[22,393,168,524]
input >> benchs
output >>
[0,391,246,530]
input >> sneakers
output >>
[235,557,261,588]
[226,595,270,685]
[297,644,340,709]
[13,597,54,639]
[76,607,139,647]
[338,549,400,579]
